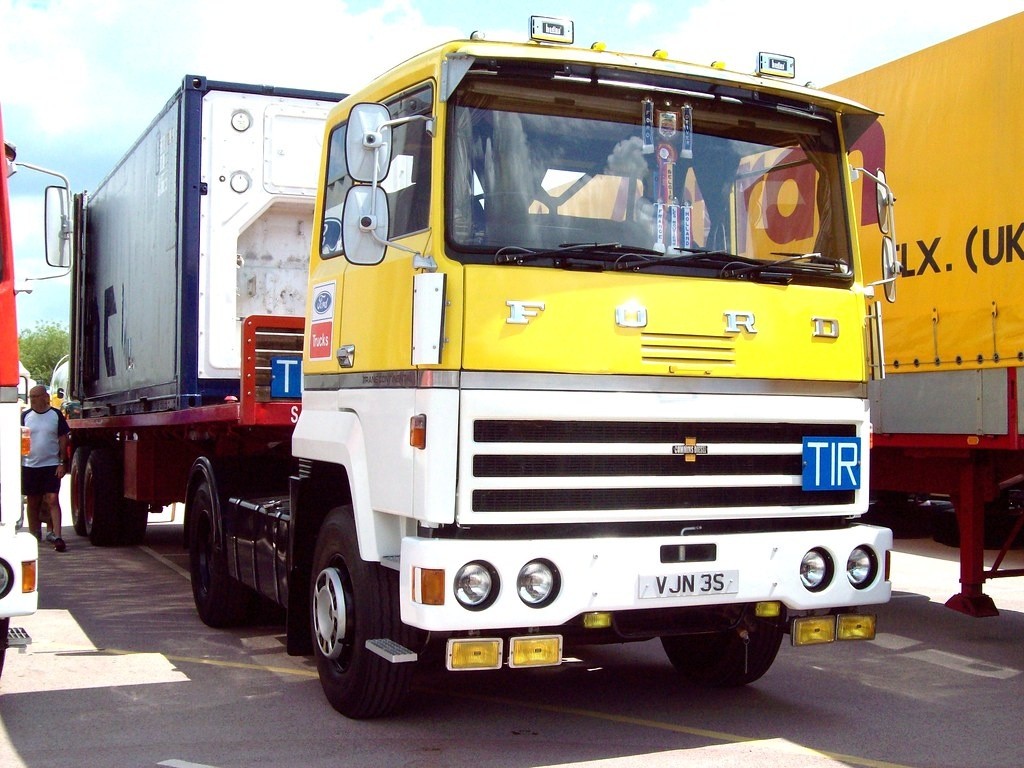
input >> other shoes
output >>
[46,532,55,541]
[54,539,66,551]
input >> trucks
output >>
[48,355,68,419]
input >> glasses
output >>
[29,392,45,398]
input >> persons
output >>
[20,385,71,552]
[39,392,69,542]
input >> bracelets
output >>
[59,460,64,465]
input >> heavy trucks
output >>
[0,105,75,676]
[62,16,912,721]
[535,9,1024,618]
